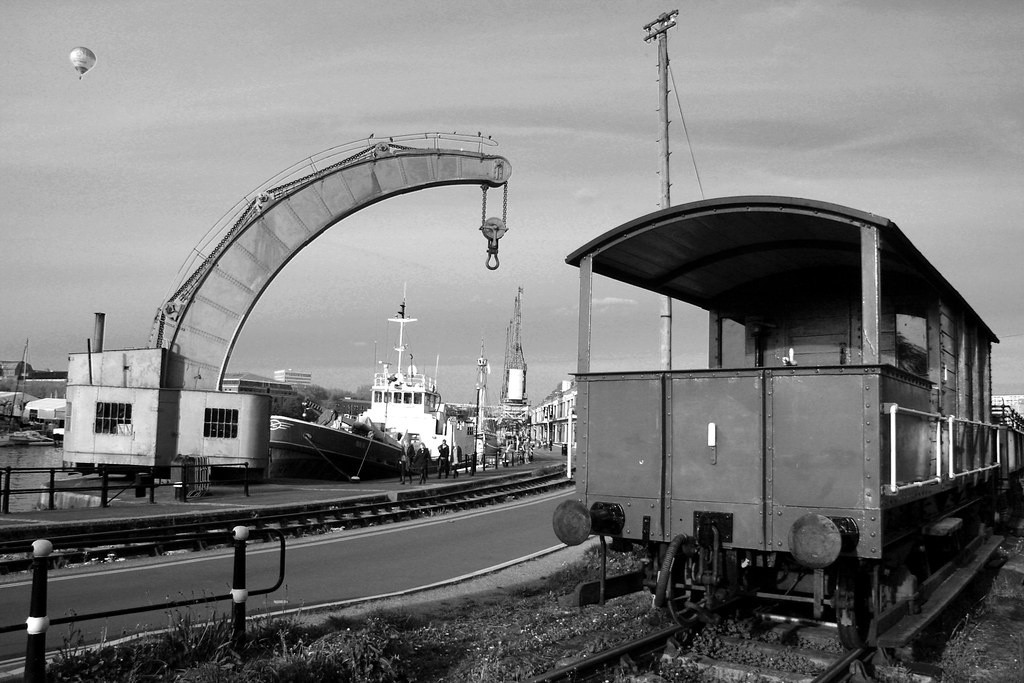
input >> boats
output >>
[326,276,533,473]
[268,416,405,479]
[0,335,65,450]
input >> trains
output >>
[553,193,1024,654]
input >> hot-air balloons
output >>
[71,47,97,79]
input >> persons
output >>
[398,439,415,484]
[437,440,449,479]
[450,441,463,479]
[414,443,434,484]
[522,439,531,464]
[544,439,553,451]
